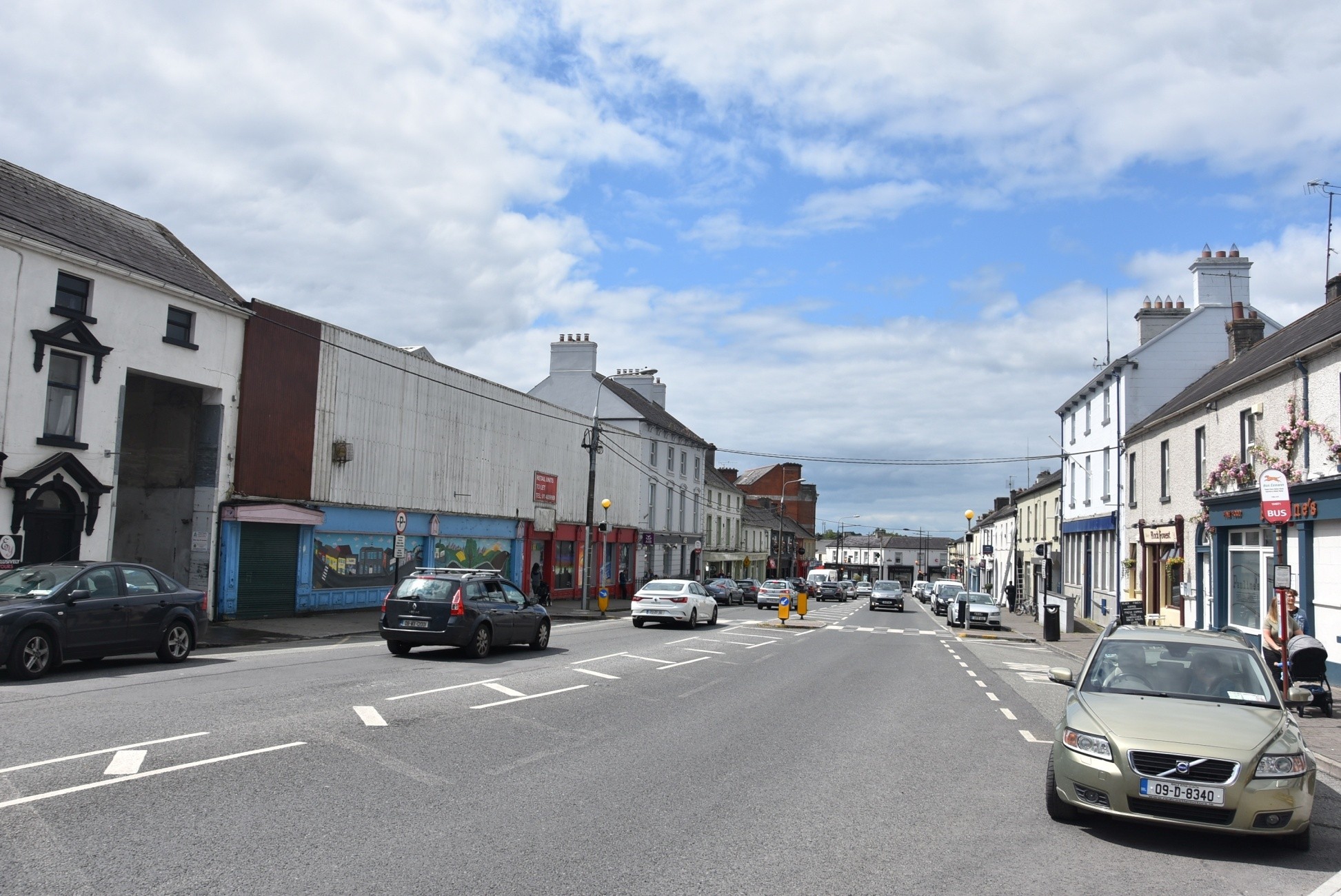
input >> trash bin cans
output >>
[1044,603,1062,642]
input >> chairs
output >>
[90,575,114,599]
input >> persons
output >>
[1194,653,1235,698]
[1004,581,1016,613]
[718,569,732,579]
[1274,661,1290,680]
[1262,596,1304,690]
[619,569,627,599]
[531,562,543,598]
[1285,590,1310,637]
[645,569,654,583]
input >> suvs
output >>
[379,566,551,659]
[784,576,810,600]
[868,579,907,613]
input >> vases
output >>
[1172,563,1179,569]
[1127,563,1133,568]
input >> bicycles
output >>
[1015,595,1038,617]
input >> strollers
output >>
[1280,634,1333,718]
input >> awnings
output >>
[767,558,776,569]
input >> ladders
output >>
[1013,565,1025,613]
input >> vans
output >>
[930,580,967,616]
[807,569,838,595]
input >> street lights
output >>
[776,478,807,579]
[581,368,658,609]
[835,515,860,568]
[902,526,925,580]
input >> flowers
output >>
[1165,557,1184,581]
[1191,397,1341,534]
[1121,559,1136,576]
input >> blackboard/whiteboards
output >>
[1120,601,1146,626]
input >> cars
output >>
[911,580,935,604]
[947,591,1001,631]
[1045,618,1318,849]
[856,582,873,597]
[698,578,745,607]
[815,581,848,603]
[805,580,820,598]
[839,572,868,600]
[732,579,762,603]
[631,579,718,630]
[757,579,801,611]
[0,560,210,681]
